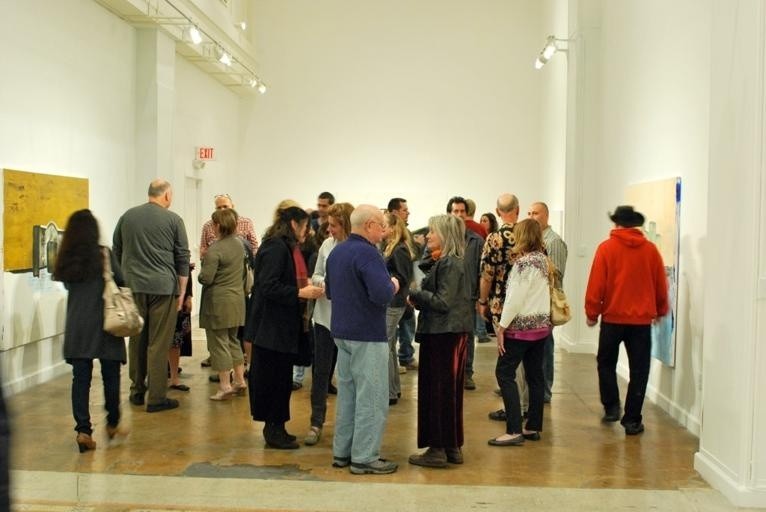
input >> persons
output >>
[584,204,668,436]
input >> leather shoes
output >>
[170,383,192,393]
[601,413,619,423]
[524,431,541,441]
[487,432,525,448]
[127,392,146,406]
[303,427,323,445]
[488,407,504,420]
[145,397,179,413]
[500,411,526,424]
[623,419,646,435]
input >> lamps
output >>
[530,32,572,73]
[159,22,268,96]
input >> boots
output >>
[281,422,297,441]
[263,422,299,452]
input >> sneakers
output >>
[290,380,303,391]
[463,377,476,390]
[327,382,338,395]
[406,359,420,371]
[404,447,448,468]
[389,398,396,406]
[448,444,466,464]
[350,458,399,475]
[166,364,184,375]
[332,455,351,468]
[397,391,402,399]
[477,336,493,344]
[200,356,211,368]
[209,373,233,382]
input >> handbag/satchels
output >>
[101,283,147,337]
[244,260,255,297]
[548,257,573,326]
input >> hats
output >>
[609,205,648,227]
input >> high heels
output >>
[232,382,248,392]
[74,433,98,452]
[209,387,238,401]
[104,424,117,440]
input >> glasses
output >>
[215,194,232,202]
[371,221,388,229]
